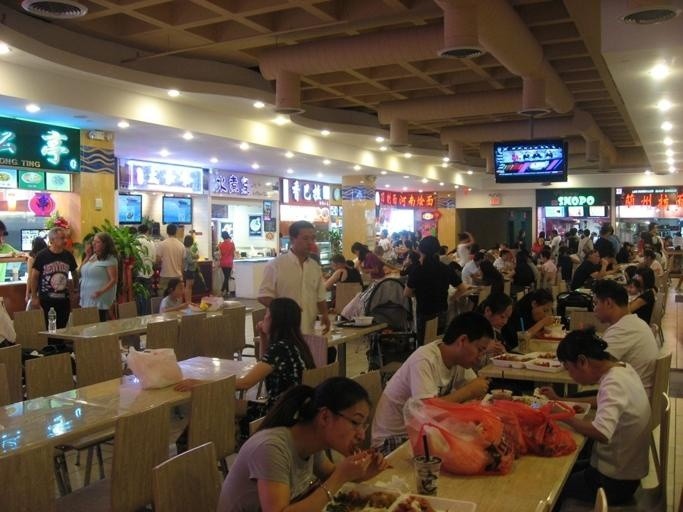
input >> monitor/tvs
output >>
[20,229,52,252]
[493,140,568,182]
[162,196,192,225]
[119,194,143,224]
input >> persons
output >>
[471,290,523,397]
[1,222,28,346]
[78,232,121,321]
[311,221,681,346]
[538,325,655,511]
[368,311,494,457]
[256,220,331,336]
[30,226,80,344]
[173,296,317,452]
[125,224,236,315]
[500,287,556,352]
[590,279,659,407]
[214,375,397,511]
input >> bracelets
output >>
[318,481,334,502]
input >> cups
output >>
[490,388,513,400]
[516,331,531,353]
[413,455,442,496]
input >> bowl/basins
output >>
[353,316,374,325]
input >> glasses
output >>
[336,413,370,433]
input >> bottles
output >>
[48,307,56,335]
